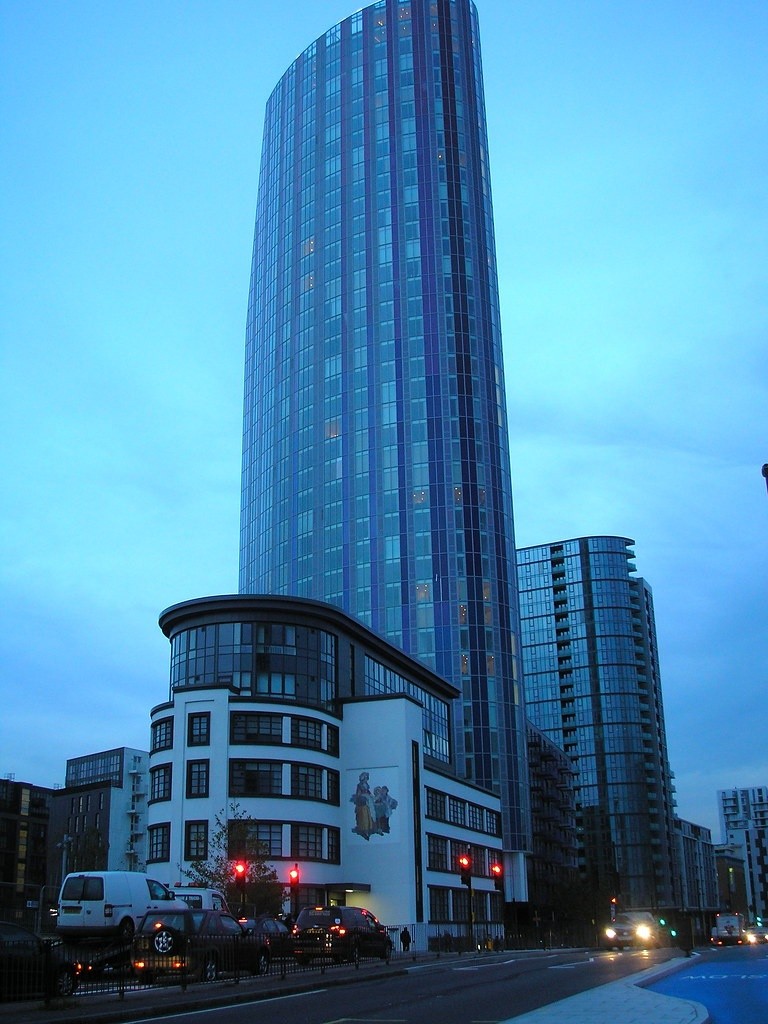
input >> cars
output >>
[607,911,661,949]
[281,905,393,963]
[709,926,768,945]
[0,919,78,1002]
[236,918,290,950]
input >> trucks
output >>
[715,913,745,945]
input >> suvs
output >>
[129,908,272,981]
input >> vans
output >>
[53,871,190,940]
[53,885,233,974]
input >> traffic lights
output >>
[460,855,472,885]
[289,868,299,895]
[234,860,245,890]
[492,865,502,890]
